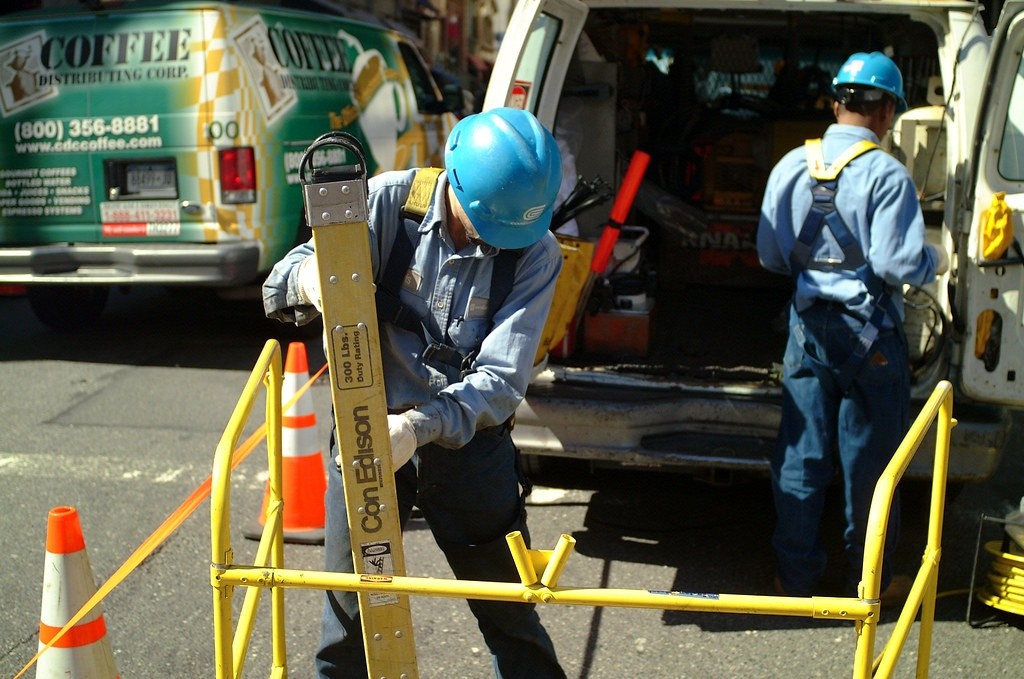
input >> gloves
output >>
[334,410,420,477]
[295,247,325,309]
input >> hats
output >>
[441,104,566,251]
[830,50,910,112]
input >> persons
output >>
[260,107,566,678]
[754,52,952,610]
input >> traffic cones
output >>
[33,505,128,679]
[241,340,329,548]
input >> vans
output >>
[0,0,478,288]
[447,0,1020,478]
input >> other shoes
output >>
[875,574,917,611]
